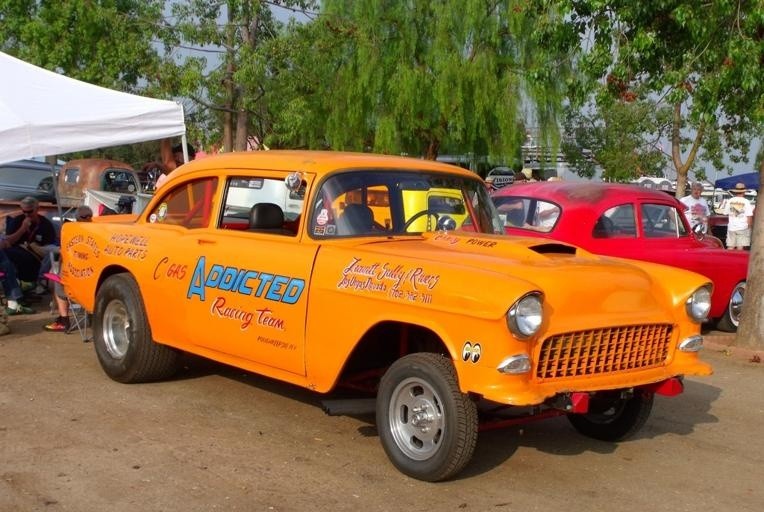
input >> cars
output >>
[463,180,752,334]
[59,148,714,482]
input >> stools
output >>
[42,273,94,342]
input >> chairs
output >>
[590,213,616,239]
[332,203,385,240]
[245,201,287,235]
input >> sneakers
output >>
[8,305,33,314]
[44,316,70,331]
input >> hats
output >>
[20,242,42,261]
[730,184,751,193]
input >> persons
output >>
[155,142,196,190]
[722,181,753,252]
[668,182,713,238]
[42,204,95,333]
[536,176,565,228]
[6,197,55,296]
[115,195,136,215]
[497,171,532,225]
[520,168,536,183]
[0,236,37,315]
[470,176,494,208]
